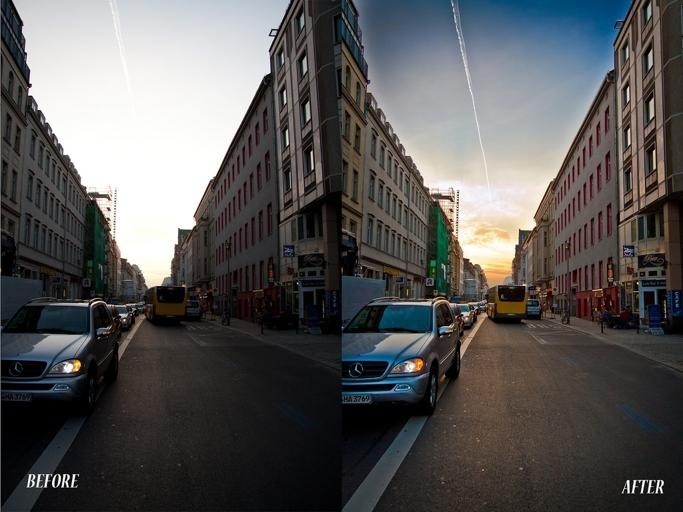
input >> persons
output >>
[256,301,288,328]
[593,306,632,329]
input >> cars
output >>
[106,301,145,330]
[448,301,487,327]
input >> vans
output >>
[527,299,541,315]
[186,300,201,318]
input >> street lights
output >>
[565,242,570,324]
[223,243,232,323]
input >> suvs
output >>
[340,296,460,416]
[1,296,119,417]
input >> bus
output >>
[144,286,187,322]
[485,285,528,319]
